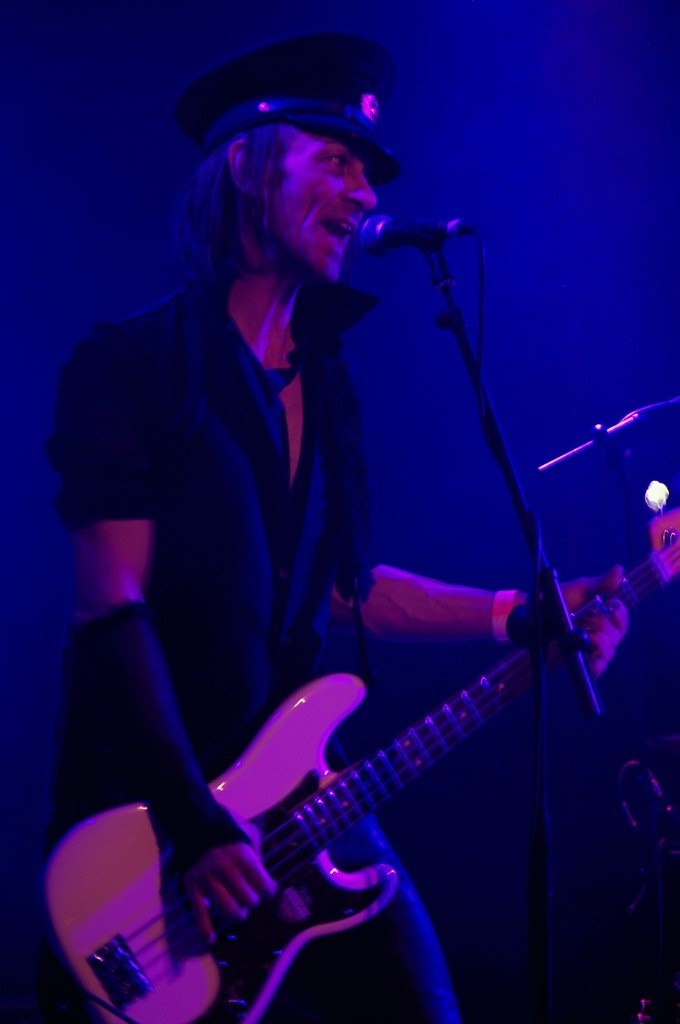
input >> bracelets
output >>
[492,590,519,641]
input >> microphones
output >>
[354,212,474,260]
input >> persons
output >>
[43,29,631,1024]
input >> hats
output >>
[173,30,403,186]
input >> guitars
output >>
[37,476,678,1024]
